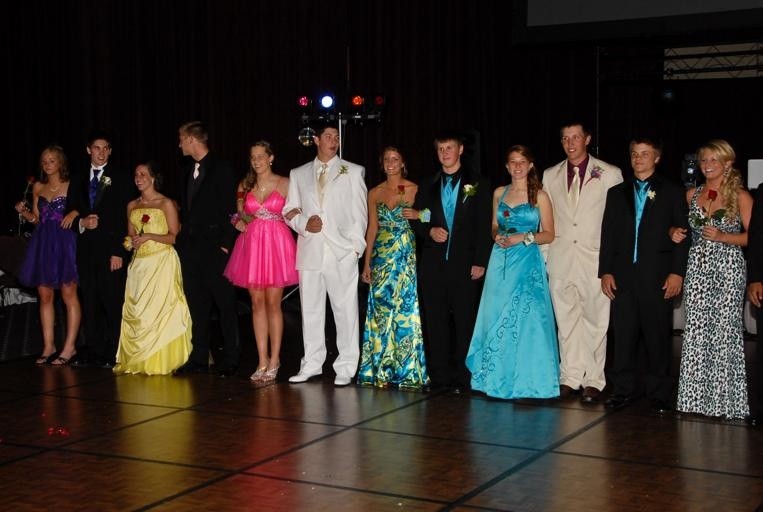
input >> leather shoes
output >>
[603,393,637,410]
[556,384,573,401]
[73,354,90,367]
[334,374,352,386]
[650,400,673,416]
[747,414,761,425]
[580,387,601,404]
[288,367,323,383]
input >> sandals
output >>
[36,351,57,364]
[52,353,79,366]
[251,362,281,381]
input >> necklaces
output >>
[258,183,268,192]
[140,195,153,205]
[48,182,60,192]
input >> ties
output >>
[89,169,102,210]
[444,177,453,206]
[637,181,648,203]
[319,164,328,188]
[193,163,200,179]
[567,167,580,217]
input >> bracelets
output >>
[28,213,36,224]
[522,230,536,247]
[67,133,132,368]
[297,207,302,214]
[418,208,431,224]
[228,211,242,225]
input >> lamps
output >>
[296,92,386,148]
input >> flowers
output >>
[18,175,35,235]
[128,213,151,271]
[94,175,112,213]
[460,182,480,205]
[331,163,349,184]
[647,188,657,205]
[685,189,726,227]
[503,210,510,282]
[584,164,605,188]
[230,209,253,233]
[395,184,410,209]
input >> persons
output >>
[39,363,85,442]
[282,123,367,385]
[746,183,761,428]
[408,128,488,393]
[597,138,692,415]
[112,162,193,376]
[172,120,243,379]
[539,120,623,403]
[669,139,753,419]
[15,147,80,368]
[222,140,297,382]
[464,145,562,403]
[358,145,431,389]
[249,380,299,512]
